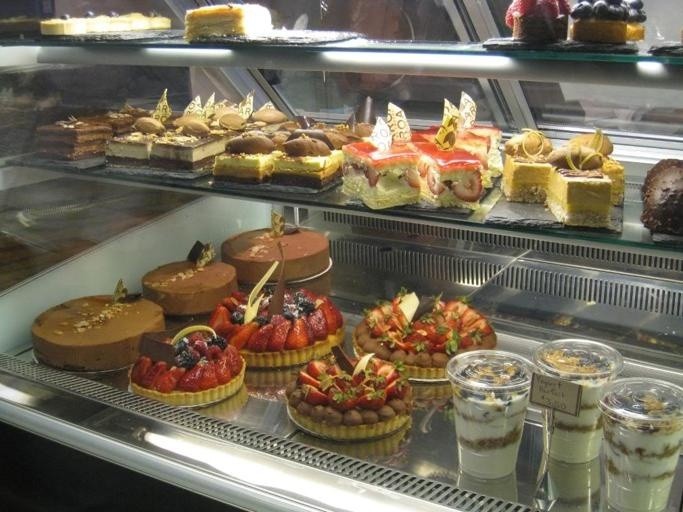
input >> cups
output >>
[544,452,605,511]
[534,337,628,468]
[442,350,536,482]
[592,375,683,511]
[456,470,519,504]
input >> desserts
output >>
[36,87,626,227]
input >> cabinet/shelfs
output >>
[0,27,680,511]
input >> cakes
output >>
[30,278,166,373]
[285,345,415,443]
[352,288,499,378]
[183,4,270,39]
[570,1,630,43]
[141,242,240,316]
[207,241,346,369]
[1,12,38,35]
[1,86,90,156]
[129,323,247,407]
[38,11,172,37]
[627,1,647,41]
[505,1,570,40]
[641,157,683,236]
[221,210,330,283]
